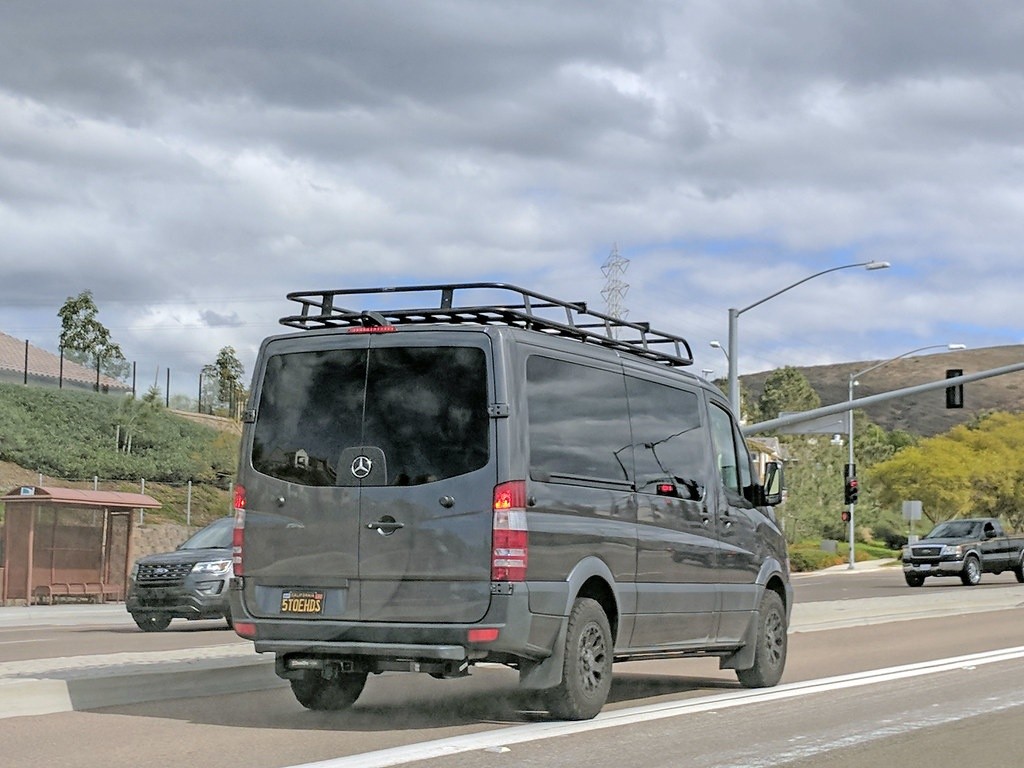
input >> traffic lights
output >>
[847,477,860,507]
[842,511,851,523]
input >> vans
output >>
[233,282,791,723]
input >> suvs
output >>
[123,514,236,633]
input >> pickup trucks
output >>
[899,518,1024,587]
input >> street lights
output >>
[708,341,740,423]
[726,259,892,441]
[846,343,967,572]
